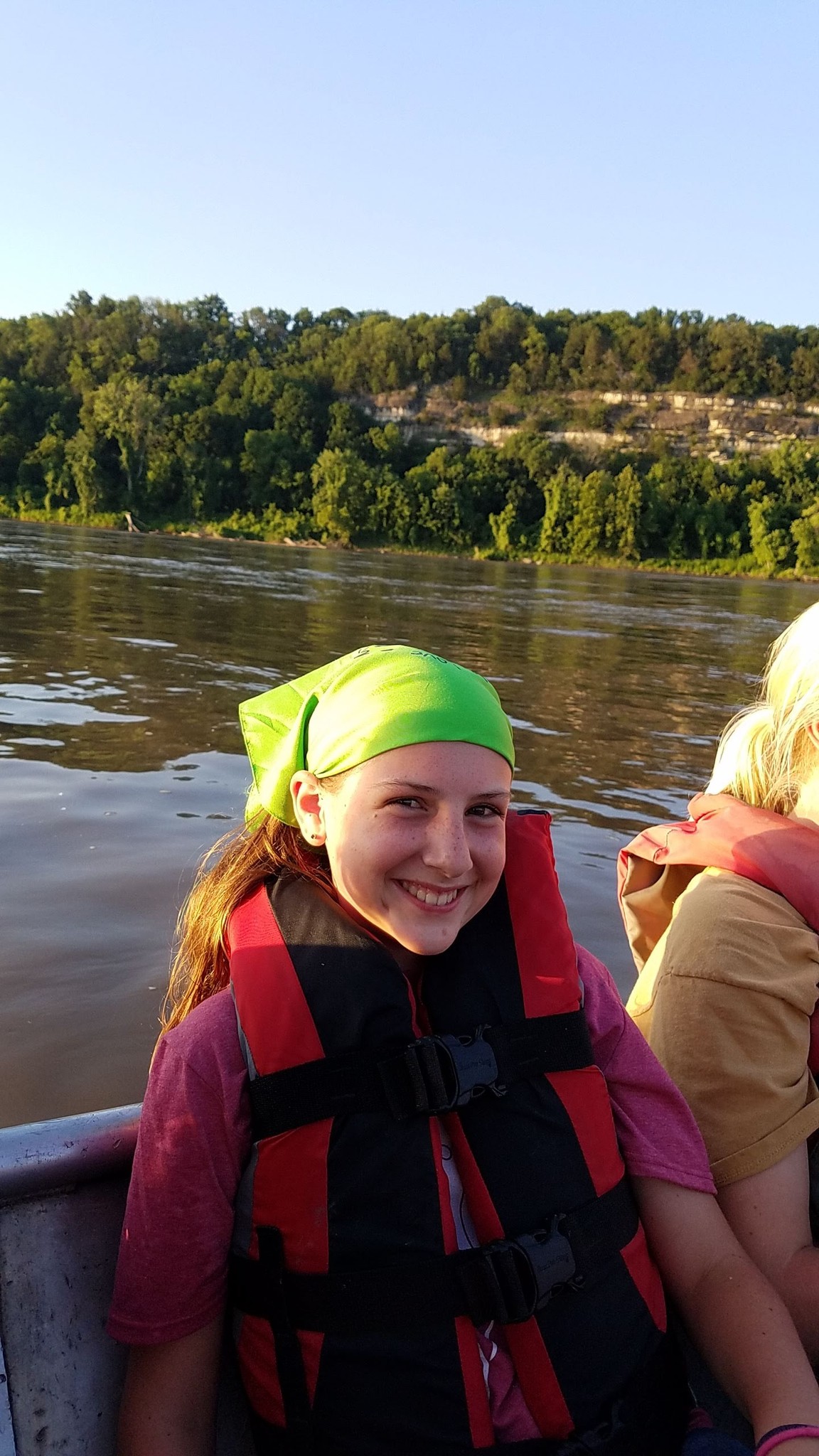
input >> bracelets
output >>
[756,1424,819,1456]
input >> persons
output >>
[107,643,819,1456]
[618,601,819,1381]
